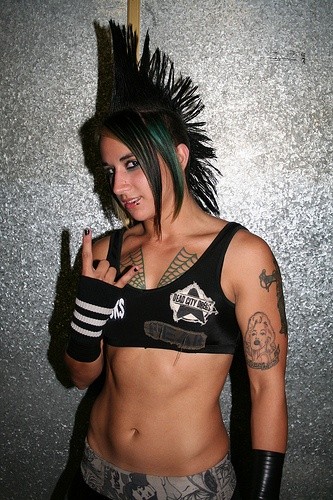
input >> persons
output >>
[54,17,290,500]
